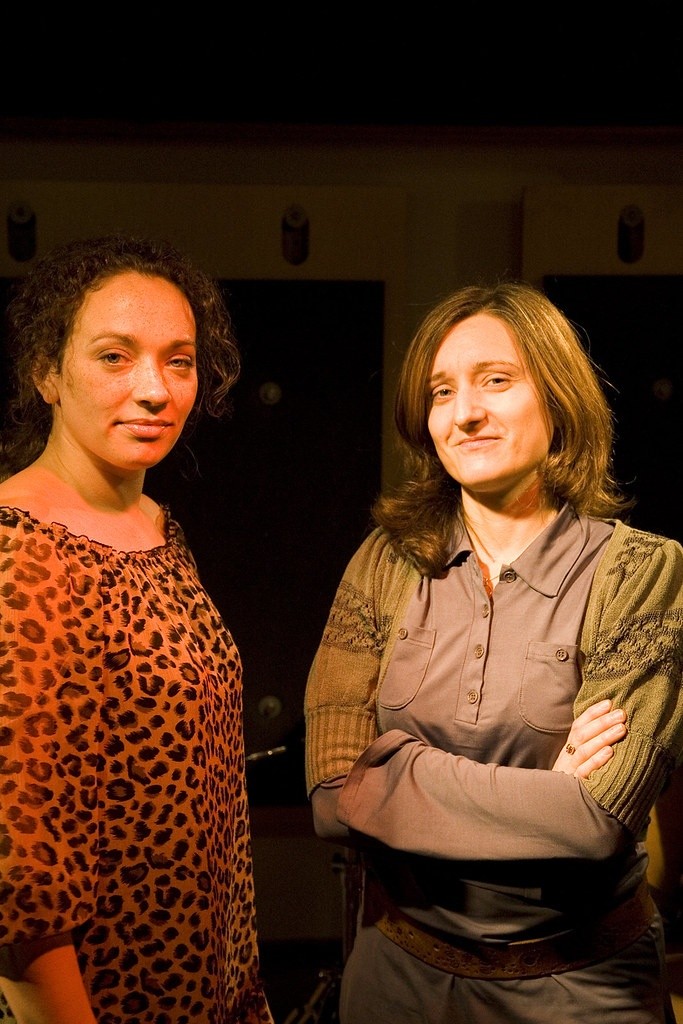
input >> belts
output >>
[364,875,654,979]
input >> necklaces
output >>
[482,573,500,596]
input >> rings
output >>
[565,743,576,754]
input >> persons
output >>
[305,284,682,1024]
[0,237,278,1023]
